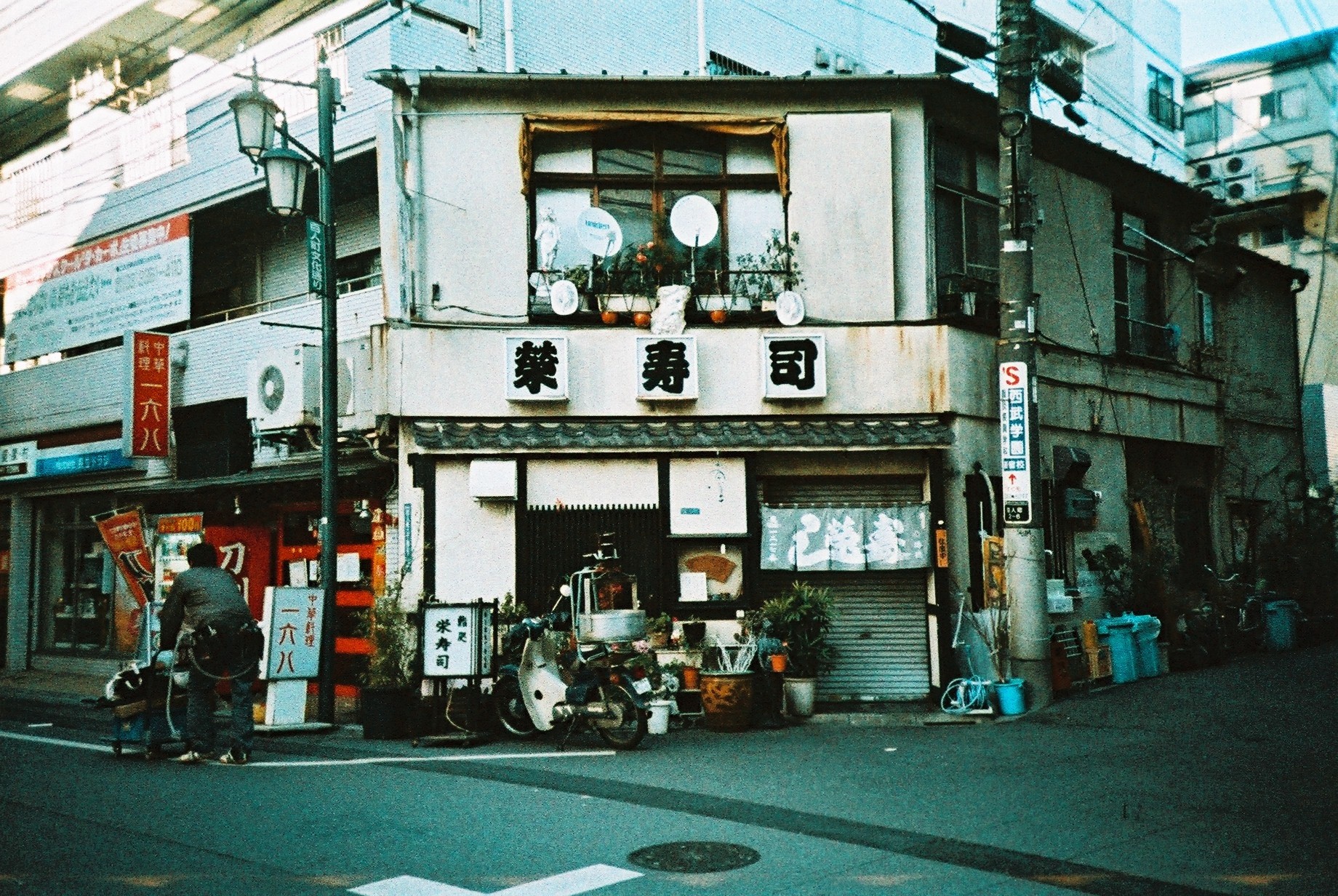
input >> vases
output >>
[634,312,651,326]
[694,669,755,727]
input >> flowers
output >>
[635,240,672,298]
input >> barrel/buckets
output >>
[644,701,671,734]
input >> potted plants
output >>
[681,651,703,689]
[352,583,414,741]
[643,611,673,648]
[758,581,839,717]
[682,615,706,648]
[702,247,727,322]
[596,245,635,323]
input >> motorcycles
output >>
[491,584,653,751]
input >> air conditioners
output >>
[249,344,322,429]
[1194,156,1256,203]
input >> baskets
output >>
[1208,579,1234,602]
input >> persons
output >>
[157,544,265,765]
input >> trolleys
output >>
[113,643,193,764]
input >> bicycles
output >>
[1195,563,1264,650]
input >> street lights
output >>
[227,45,348,726]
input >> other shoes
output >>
[167,750,201,763]
[220,747,246,763]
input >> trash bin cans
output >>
[1127,612,1162,678]
[1261,600,1299,652]
[1094,611,1138,683]
